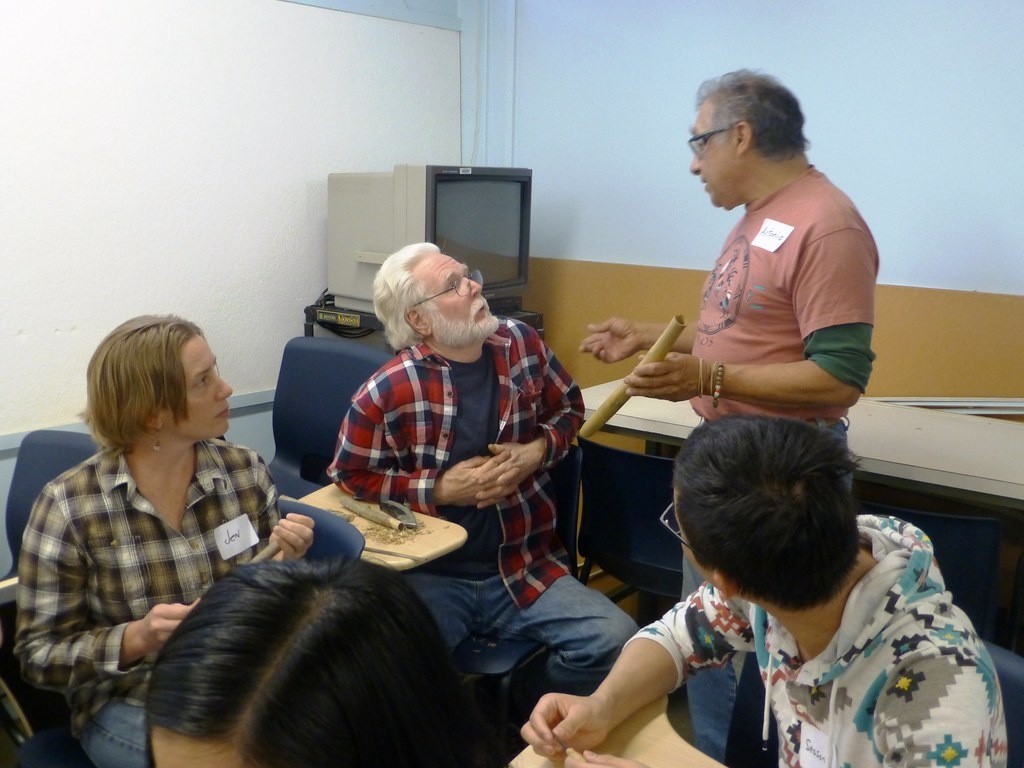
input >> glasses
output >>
[661,503,704,560]
[413,270,483,309]
[686,127,730,158]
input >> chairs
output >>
[274,497,365,563]
[451,445,585,747]
[851,496,1000,639]
[0,429,100,580]
[981,640,1024,768]
[570,432,683,631]
[265,334,398,506]
[0,576,98,767]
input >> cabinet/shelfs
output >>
[302,291,546,378]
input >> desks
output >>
[299,477,468,572]
[579,375,1024,508]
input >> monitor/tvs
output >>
[326,163,532,315]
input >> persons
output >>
[579,68,879,764]
[143,561,474,768]
[15,315,315,768]
[327,242,640,696]
[521,413,1007,768]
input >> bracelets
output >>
[710,361,724,408]
[698,358,703,398]
